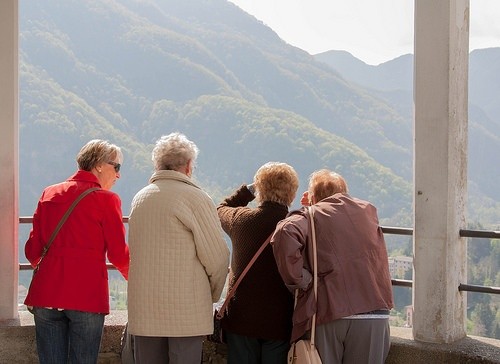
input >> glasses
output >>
[107,161,121,172]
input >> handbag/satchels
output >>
[26,268,39,313]
[287,339,323,364]
[207,300,225,344]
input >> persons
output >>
[24,139,132,364]
[126,132,231,364]
[216,160,295,364]
[270,168,395,364]
